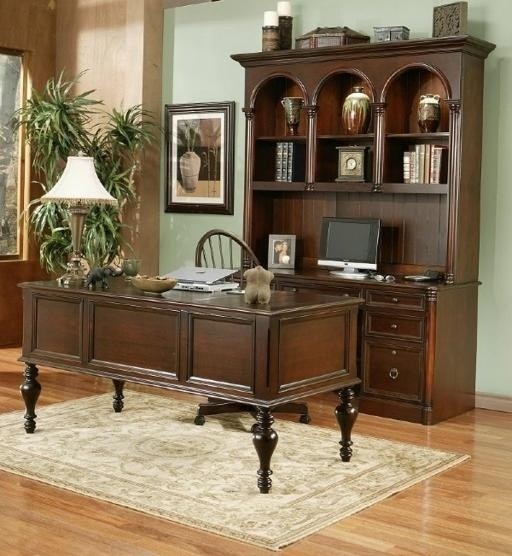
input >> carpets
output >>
[0,387,470,554]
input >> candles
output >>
[262,12,280,26]
[277,1,292,17]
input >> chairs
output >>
[194,229,312,426]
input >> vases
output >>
[179,151,201,193]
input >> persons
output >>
[279,241,290,264]
[273,241,283,264]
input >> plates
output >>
[405,276,430,281]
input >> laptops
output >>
[162,266,239,292]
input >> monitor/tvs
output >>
[317,217,381,280]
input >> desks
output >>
[17,280,367,494]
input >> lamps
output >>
[39,155,120,288]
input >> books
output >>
[402,142,448,185]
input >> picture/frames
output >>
[267,233,297,277]
[164,101,236,215]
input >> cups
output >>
[123,259,141,276]
